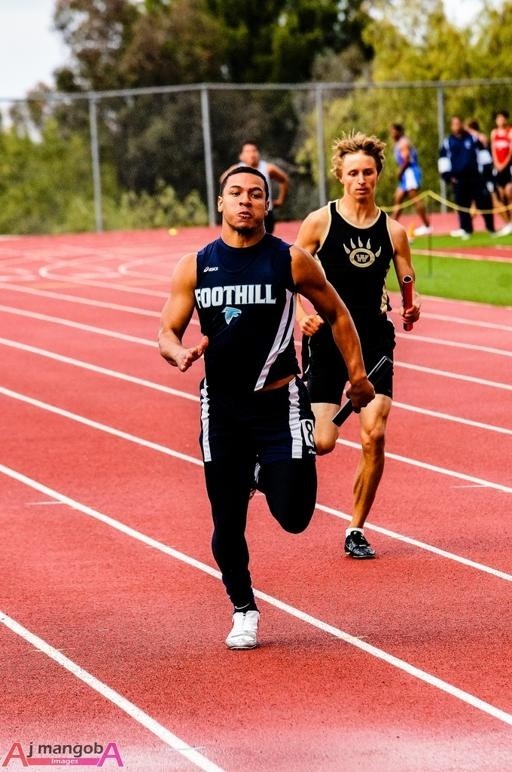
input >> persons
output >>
[387,124,432,238]
[436,109,512,242]
[291,127,425,562]
[156,160,378,651]
[216,139,290,237]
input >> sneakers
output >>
[344,531,376,559]
[224,610,261,650]
